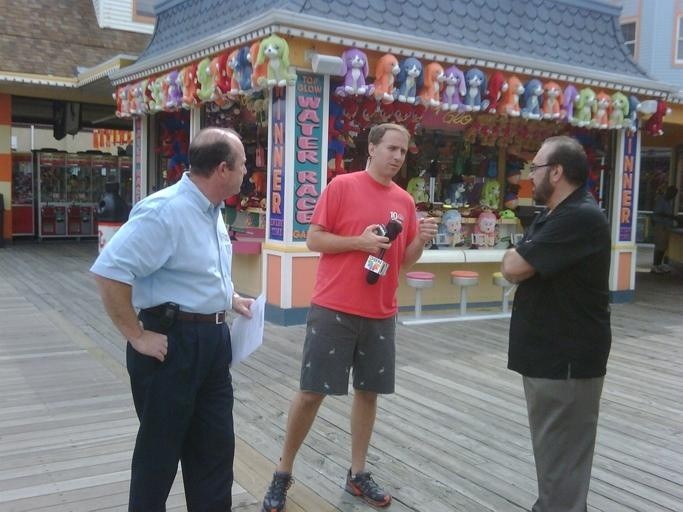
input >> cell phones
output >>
[372,224,389,236]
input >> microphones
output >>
[364,220,402,285]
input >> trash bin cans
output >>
[95,192,127,256]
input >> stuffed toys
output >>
[406,113,571,250]
[150,90,268,228]
[645,100,672,136]
[327,48,641,180]
[112,34,298,120]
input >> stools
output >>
[398,270,515,326]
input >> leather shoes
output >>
[146,307,225,325]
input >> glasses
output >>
[529,163,553,172]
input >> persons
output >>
[263,123,439,512]
[652,185,678,273]
[90,127,256,512]
[501,136,611,512]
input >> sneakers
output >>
[263,471,292,511]
[651,264,671,274]
[345,469,391,507]
